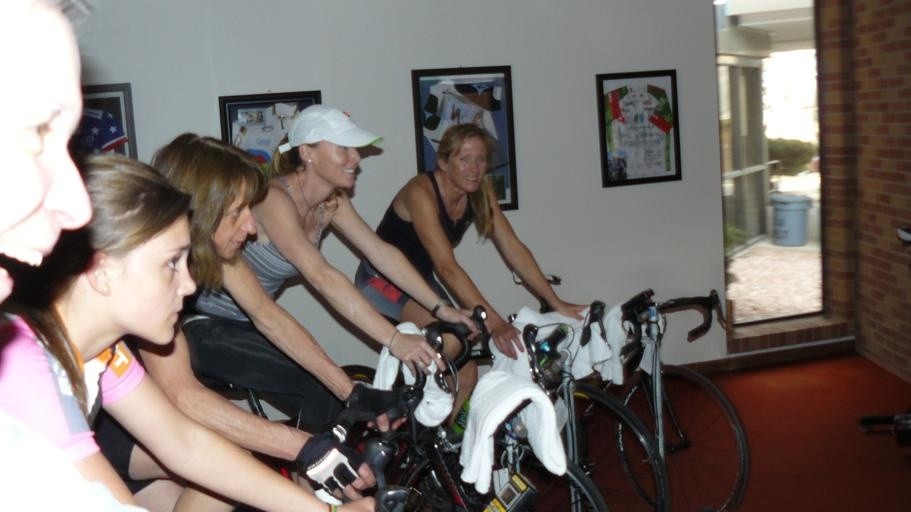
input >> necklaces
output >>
[295,171,320,213]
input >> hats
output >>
[287,103,382,149]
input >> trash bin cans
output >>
[768,194,812,246]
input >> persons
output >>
[0,155,377,511]
[92,132,408,511]
[354,123,591,426]
[179,104,481,455]
[0,2,153,512]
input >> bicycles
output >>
[190,270,752,512]
[858,410,911,444]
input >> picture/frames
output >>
[411,65,518,211]
[219,90,322,175]
[596,69,682,188]
[69,83,138,161]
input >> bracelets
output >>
[388,329,401,357]
[325,500,339,511]
[432,300,447,318]
